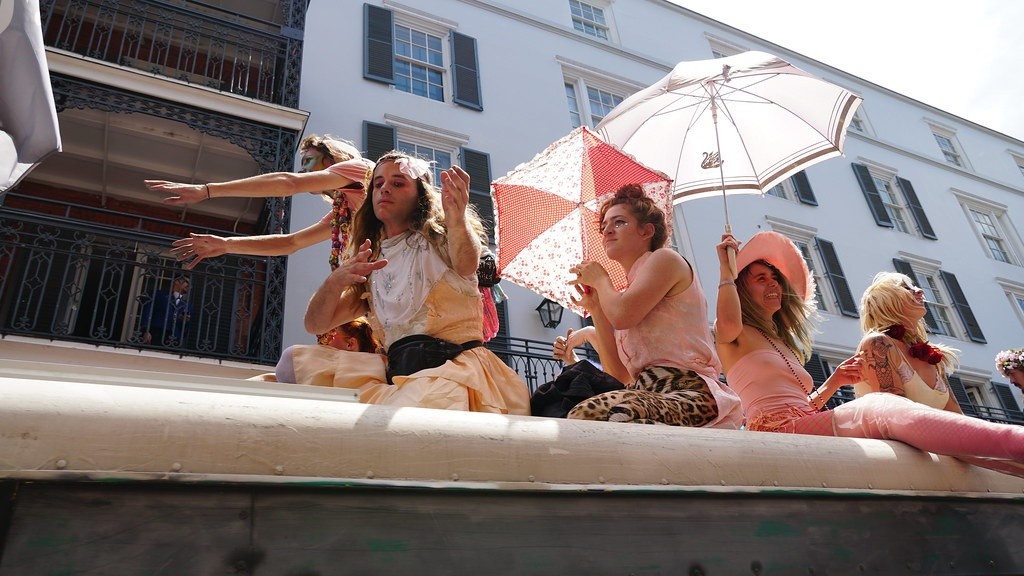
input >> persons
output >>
[851,270,1024,481]
[327,320,379,354]
[566,182,743,430]
[143,273,193,346]
[275,148,533,415]
[552,326,599,367]
[144,134,509,344]
[996,348,1024,395]
[709,236,1023,481]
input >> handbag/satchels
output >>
[385,334,483,386]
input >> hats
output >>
[733,231,811,304]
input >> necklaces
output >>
[754,319,830,412]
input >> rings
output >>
[577,269,581,275]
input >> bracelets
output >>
[204,182,210,200]
[718,280,737,289]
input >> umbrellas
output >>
[595,51,865,282]
[487,126,676,323]
